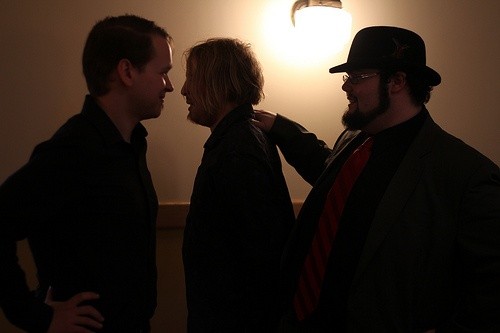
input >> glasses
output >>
[342,73,379,84]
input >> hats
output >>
[329,26,441,85]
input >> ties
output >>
[293,138,378,322]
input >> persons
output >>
[181,37,296,333]
[241,26,500,333]
[1,14,174,333]
